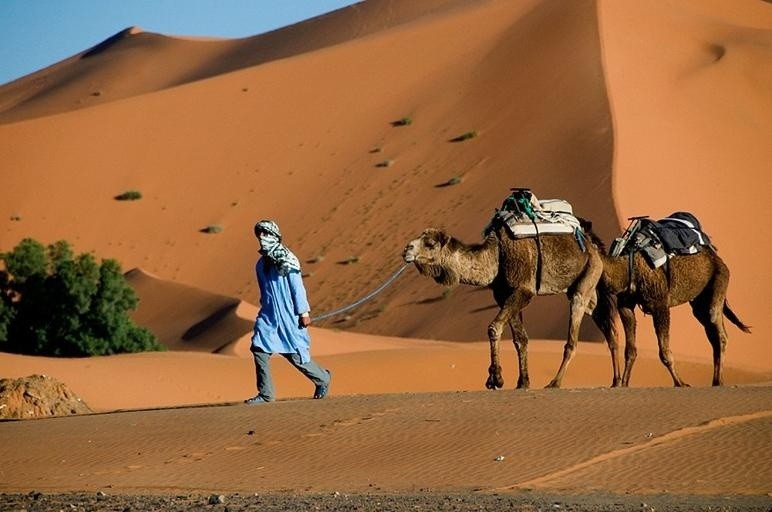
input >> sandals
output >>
[314,370,332,399]
[246,395,275,404]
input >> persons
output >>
[244,218,332,406]
